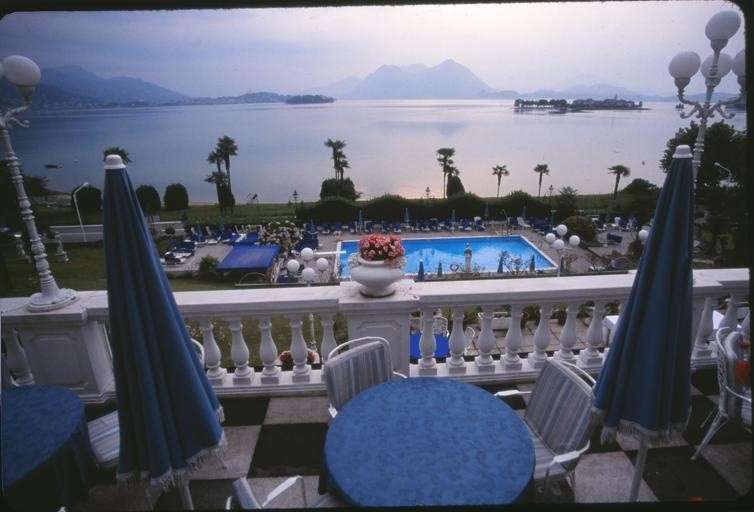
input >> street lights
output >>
[288,247,329,350]
[0,55,80,311]
[287,188,305,208]
[73,182,88,242]
[420,187,435,203]
[546,224,580,277]
[543,184,558,205]
[669,11,747,198]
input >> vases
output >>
[351,256,402,297]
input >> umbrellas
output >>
[588,142,697,503]
[99,151,227,512]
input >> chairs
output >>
[317,337,407,497]
[86,338,205,471]
[691,327,751,461]
[493,359,596,501]
[226,474,340,509]
[164,216,546,266]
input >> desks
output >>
[325,378,535,507]
[0,384,101,511]
